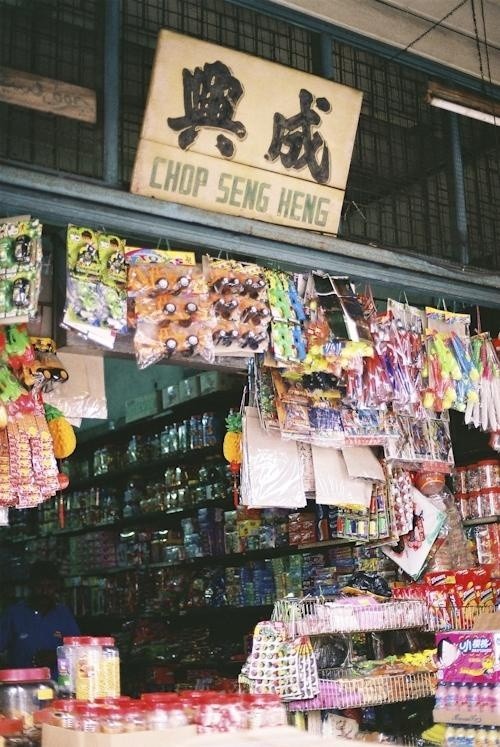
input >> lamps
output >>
[423,1,500,128]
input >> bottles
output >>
[95,413,216,523]
[1,634,284,745]
[433,680,500,747]
[452,457,499,521]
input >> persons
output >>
[0,559,81,684]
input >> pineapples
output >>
[221,412,243,466]
[44,403,77,459]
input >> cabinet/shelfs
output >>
[0,393,434,746]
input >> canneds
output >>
[0,637,288,747]
